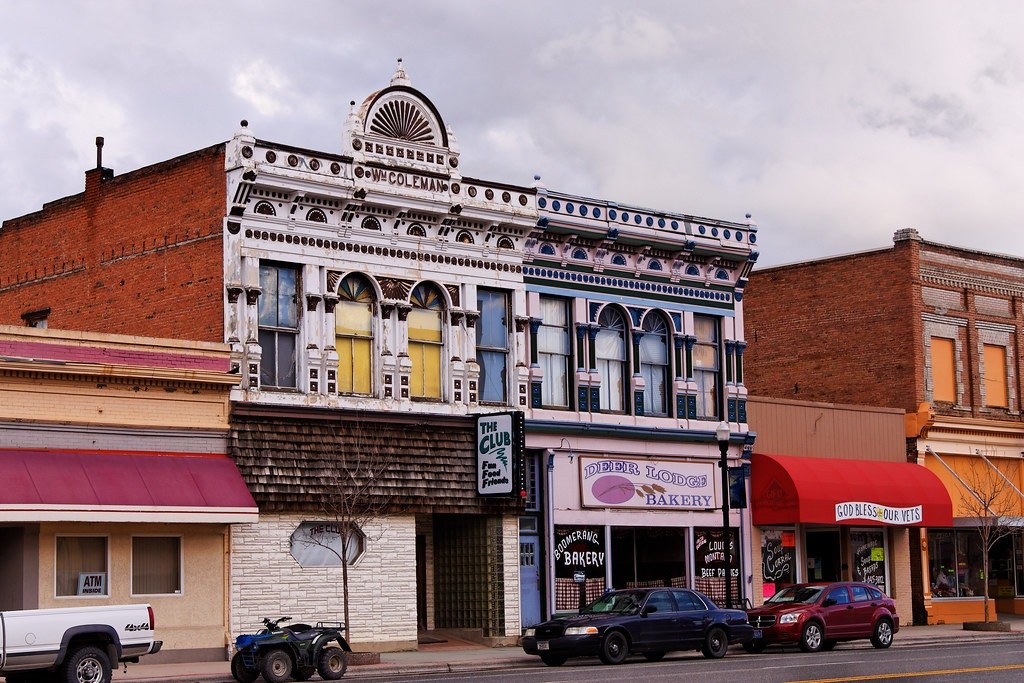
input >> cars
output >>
[521,587,755,667]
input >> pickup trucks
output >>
[0,603,164,683]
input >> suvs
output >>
[744,581,900,653]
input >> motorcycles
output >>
[230,617,353,682]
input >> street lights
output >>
[715,420,732,610]
